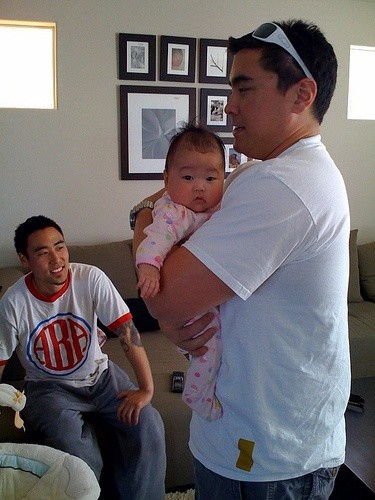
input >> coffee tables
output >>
[329,376,375,500]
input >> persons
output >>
[135,115,225,420]
[228,155,238,166]
[133,19,352,500]
[0,216,166,500]
[171,48,183,69]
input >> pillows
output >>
[348,229,364,303]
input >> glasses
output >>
[252,22,314,79]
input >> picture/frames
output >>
[200,88,233,132]
[159,35,196,82]
[119,85,196,181]
[220,137,248,179]
[118,32,156,81]
[199,37,232,83]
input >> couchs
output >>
[0,227,375,492]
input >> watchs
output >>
[129,201,153,230]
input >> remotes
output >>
[171,371,184,391]
